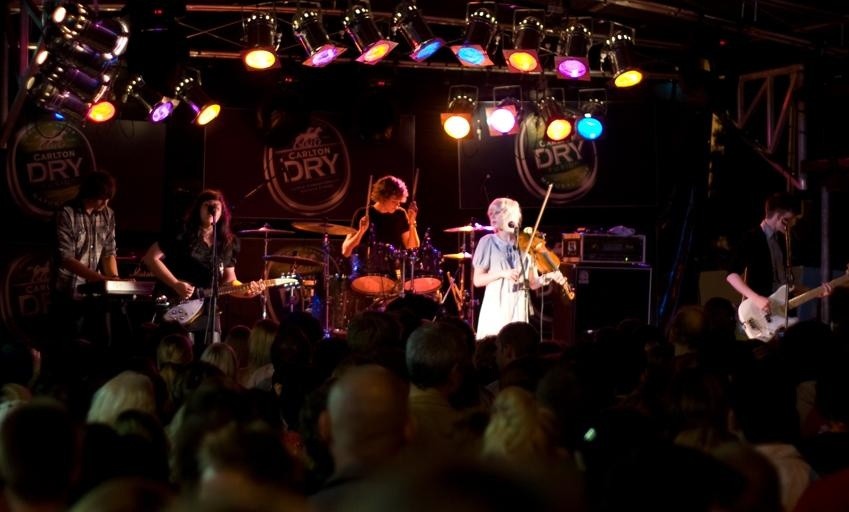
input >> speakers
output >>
[551,262,653,343]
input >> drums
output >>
[352,243,396,295]
[404,248,442,292]
[373,294,446,320]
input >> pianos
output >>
[77,279,153,297]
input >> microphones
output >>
[208,205,216,213]
[782,217,796,226]
[509,221,518,230]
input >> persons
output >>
[49,170,119,314]
[143,190,266,342]
[0,291,735,512]
[735,320,849,512]
[471,197,568,341]
[342,176,421,280]
[724,192,806,343]
[828,285,849,333]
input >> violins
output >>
[516,227,577,300]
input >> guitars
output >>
[156,269,306,327]
[738,271,849,344]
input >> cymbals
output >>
[242,225,296,234]
[292,219,358,236]
[443,222,495,232]
[444,251,472,260]
[263,251,327,267]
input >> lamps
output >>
[535,74,576,142]
[553,16,594,82]
[450,2,500,69]
[291,0,348,69]
[440,85,479,142]
[485,86,523,137]
[576,87,609,142]
[51,0,133,61]
[599,19,645,90]
[501,9,547,73]
[391,0,447,63]
[23,74,93,124]
[341,0,399,66]
[115,68,180,122]
[241,0,281,71]
[172,66,223,128]
[36,45,112,104]
